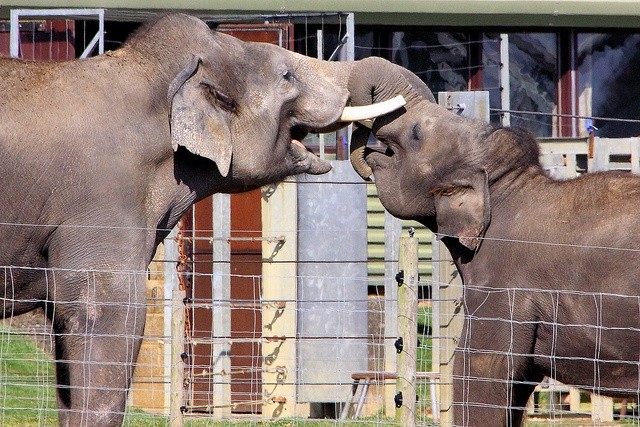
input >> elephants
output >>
[0,9,442,425]
[347,55,640,427]
